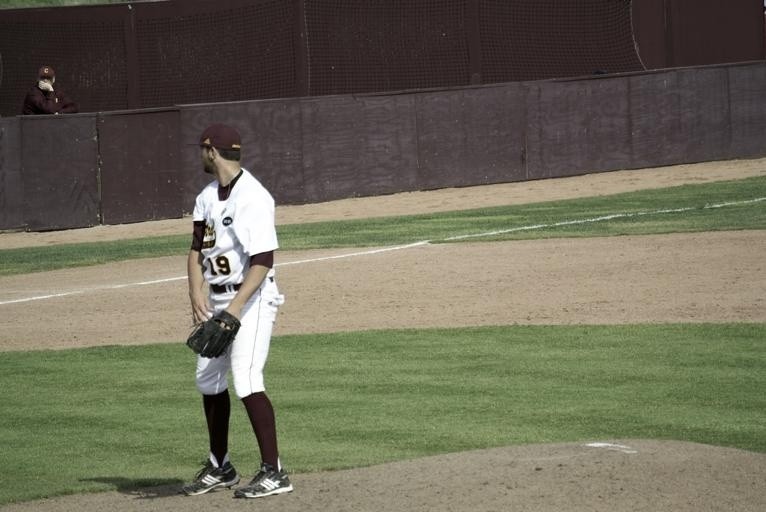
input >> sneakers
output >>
[233,463,294,498]
[182,458,240,496]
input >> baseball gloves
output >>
[186,311,241,359]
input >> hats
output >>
[198,122,241,151]
[37,65,54,80]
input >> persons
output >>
[22,64,78,116]
[181,122,294,498]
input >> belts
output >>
[210,276,274,295]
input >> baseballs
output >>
[202,311,214,320]
